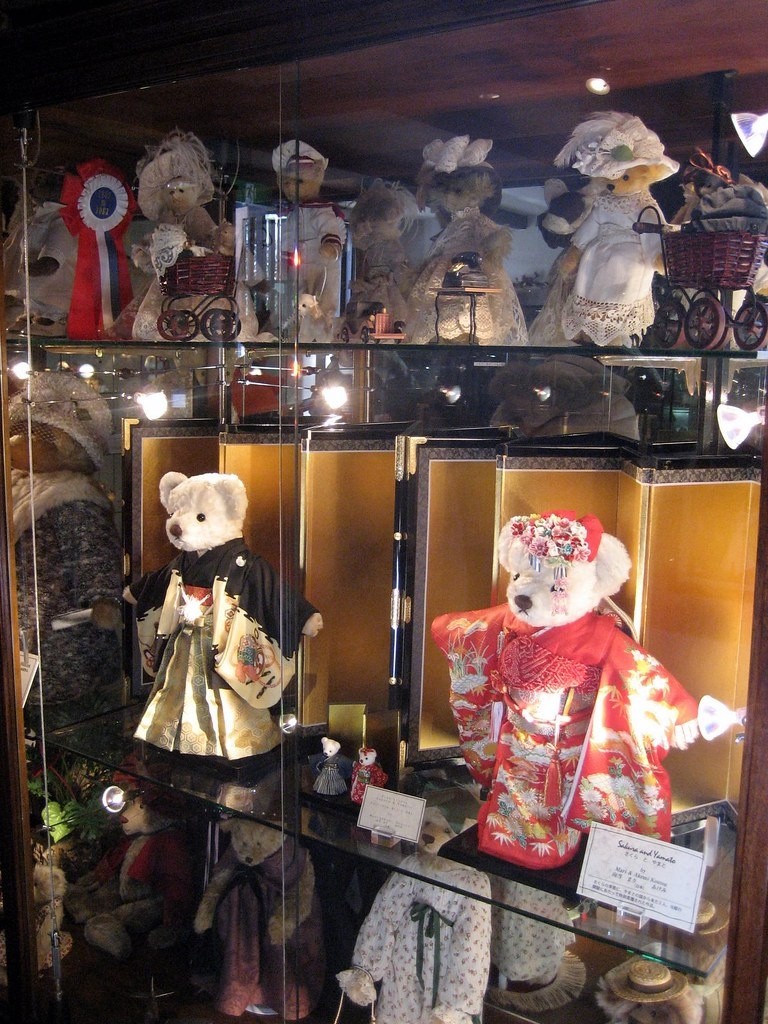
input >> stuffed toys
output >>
[263,138,343,352]
[312,737,347,796]
[489,874,581,989]
[595,952,725,1024]
[102,130,237,340]
[62,782,190,956]
[193,813,321,1018]
[663,145,767,236]
[6,373,119,711]
[335,797,493,1024]
[350,746,389,805]
[528,109,669,353]
[123,471,324,761]
[5,135,99,341]
[29,864,73,977]
[345,177,431,343]
[402,134,527,356]
[431,512,697,867]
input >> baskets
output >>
[663,230,768,289]
[159,253,237,297]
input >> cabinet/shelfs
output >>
[3,1,767,1024]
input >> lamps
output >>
[584,58,612,95]
[715,404,764,450]
[730,112,768,158]
[696,695,747,742]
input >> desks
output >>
[428,287,501,345]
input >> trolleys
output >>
[156,250,242,341]
[626,209,768,351]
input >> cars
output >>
[338,298,408,343]
